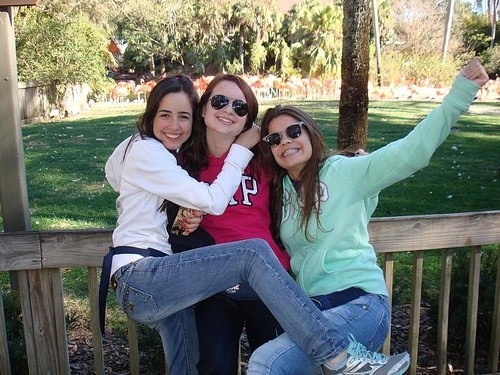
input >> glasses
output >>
[262,121,305,147]
[205,95,249,116]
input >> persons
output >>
[156,76,369,375]
[105,75,410,375]
[246,59,489,375]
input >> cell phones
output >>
[170,207,197,236]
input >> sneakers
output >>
[320,332,411,375]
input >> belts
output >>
[310,288,368,312]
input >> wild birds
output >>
[95,72,500,105]
